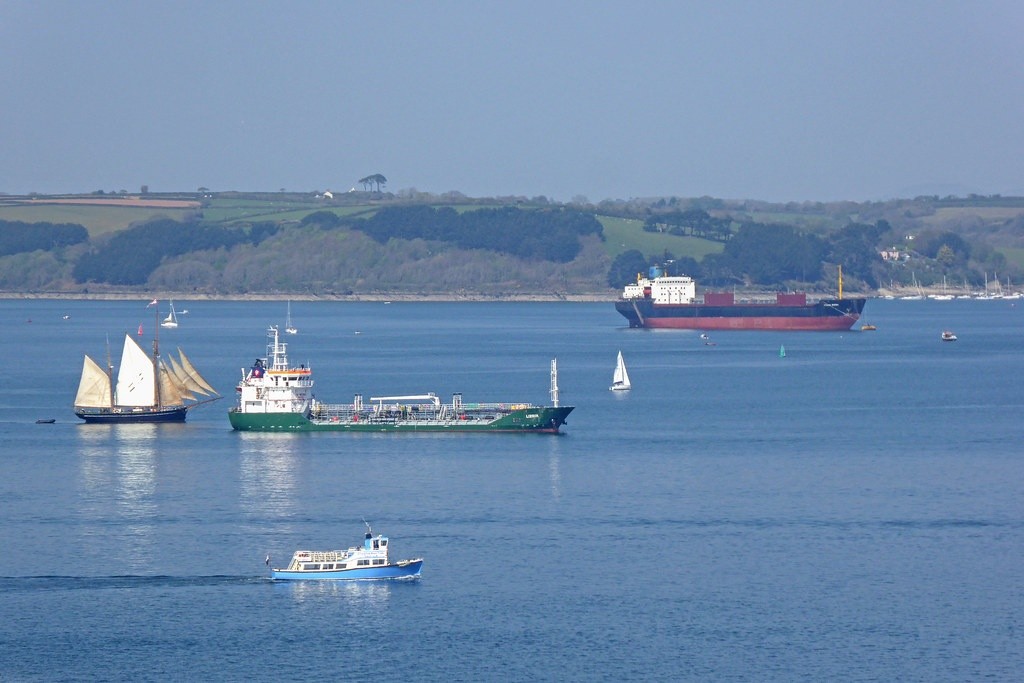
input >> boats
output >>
[36,419,56,424]
[228,324,576,433]
[942,332,958,342]
[271,516,424,580]
[614,259,866,331]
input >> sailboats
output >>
[878,271,1020,300]
[608,351,632,391]
[285,299,297,334]
[73,299,225,424]
[160,299,178,327]
